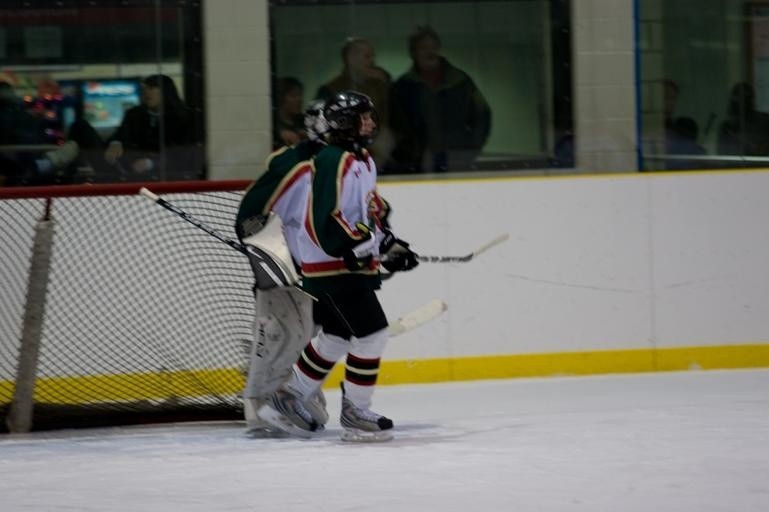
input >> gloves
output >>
[384,240,418,272]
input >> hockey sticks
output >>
[372,233,508,263]
[139,188,447,338]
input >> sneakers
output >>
[341,398,392,432]
[266,387,318,432]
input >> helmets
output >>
[324,91,376,145]
[304,101,330,147]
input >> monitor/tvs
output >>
[57,77,143,131]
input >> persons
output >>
[267,89,419,433]
[270,25,492,175]
[661,73,769,169]
[231,97,392,434]
[0,75,198,189]
